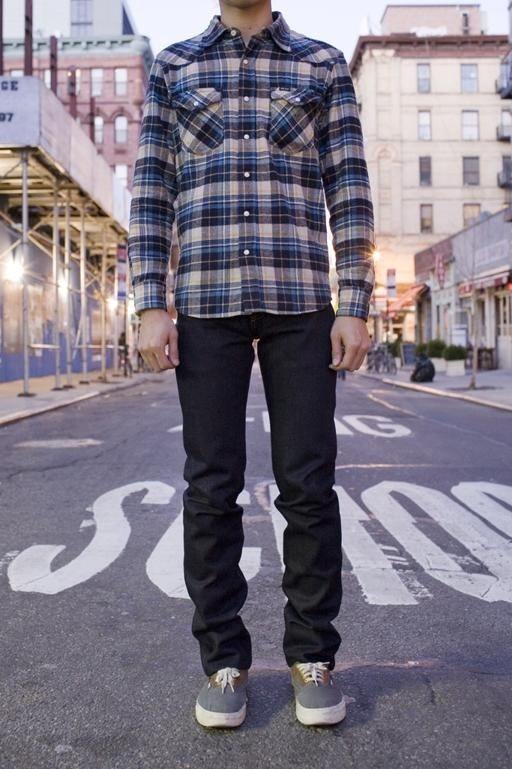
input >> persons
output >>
[126,0,375,734]
[118,331,129,367]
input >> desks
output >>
[466,346,495,370]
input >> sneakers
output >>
[291,661,346,725]
[195,667,249,727]
